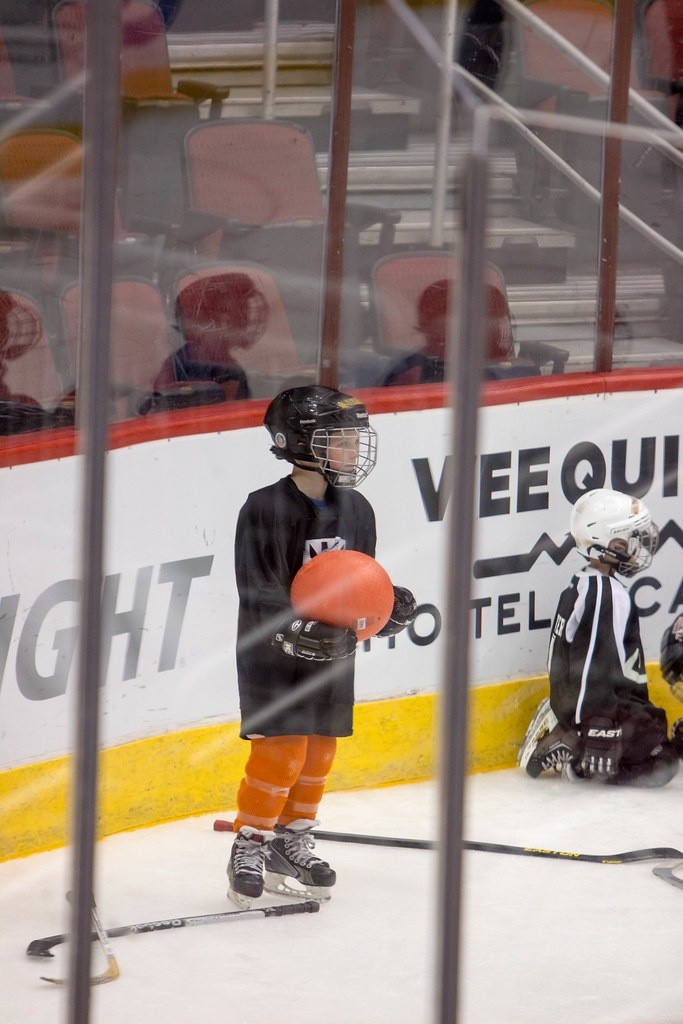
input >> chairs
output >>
[336,1,683,344]
[0,0,570,437]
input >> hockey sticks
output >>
[39,889,120,986]
[652,858,683,890]
[213,819,683,865]
[26,901,320,958]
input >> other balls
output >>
[290,550,395,641]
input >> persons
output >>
[226,386,414,912]
[514,486,682,786]
[0,279,515,437]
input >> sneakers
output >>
[262,818,336,904]
[226,825,276,909]
[516,696,581,782]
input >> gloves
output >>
[376,586,418,638]
[271,615,358,661]
[576,722,623,778]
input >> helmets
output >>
[659,613,683,686]
[263,384,378,492]
[568,487,660,577]
[176,273,255,349]
[417,279,513,362]
